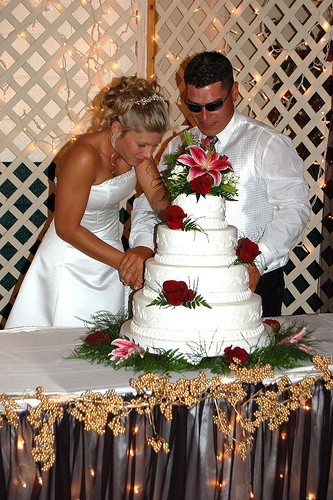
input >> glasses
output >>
[186,83,234,113]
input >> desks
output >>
[0,311,333,500]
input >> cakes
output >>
[130,193,276,365]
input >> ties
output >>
[203,136,218,153]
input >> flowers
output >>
[144,276,212,310]
[153,132,240,202]
[60,305,322,375]
[227,231,262,269]
[144,205,207,236]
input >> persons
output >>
[119,52,310,317]
[4,79,173,329]
